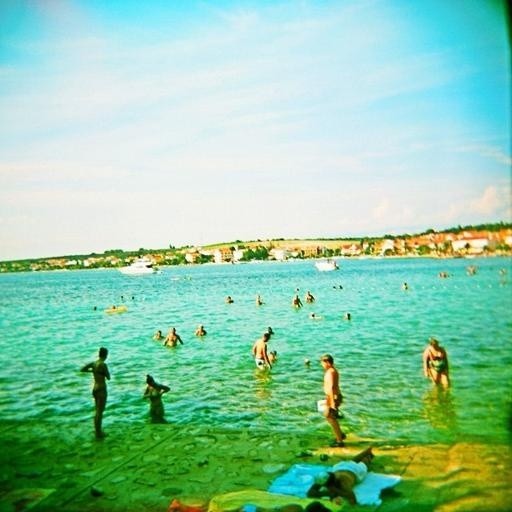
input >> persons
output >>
[292,295,304,309]
[305,446,375,505]
[227,296,233,304]
[195,324,207,336]
[163,327,183,347]
[424,337,449,389]
[402,282,409,290]
[80,347,111,437]
[256,295,262,305]
[153,330,163,340]
[253,326,278,371]
[306,290,316,304]
[320,354,347,447]
[143,374,170,424]
[346,313,350,320]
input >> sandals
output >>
[329,434,346,447]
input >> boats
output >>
[115,259,160,275]
[314,261,340,272]
